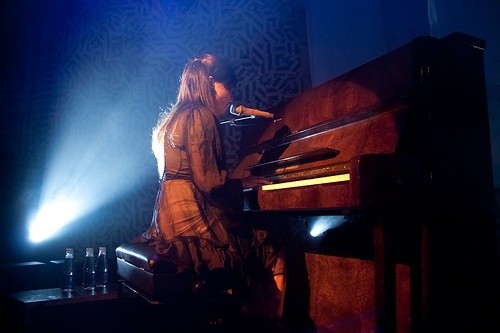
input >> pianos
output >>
[228,32,500,333]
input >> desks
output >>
[10,288,117,333]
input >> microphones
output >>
[229,104,274,118]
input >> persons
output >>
[151,52,288,333]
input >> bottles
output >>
[94,247,108,288]
[82,247,96,290]
[61,248,75,292]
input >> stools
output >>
[116,244,209,333]
